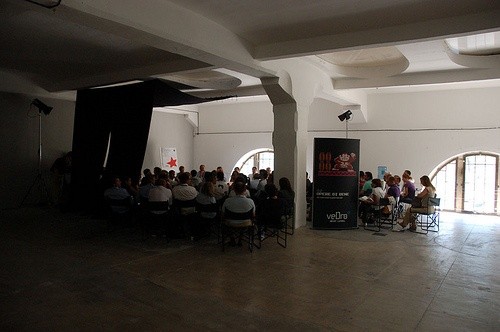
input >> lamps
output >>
[338,110,352,121]
[32,98,53,116]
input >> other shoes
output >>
[397,222,406,227]
[407,225,416,232]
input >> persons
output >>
[307,170,435,231]
[50,154,295,247]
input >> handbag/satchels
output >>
[412,197,421,208]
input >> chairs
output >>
[222,206,254,252]
[107,196,133,235]
[195,201,221,246]
[409,197,440,235]
[363,196,396,232]
[254,201,287,249]
[174,199,197,238]
[145,200,171,239]
[280,196,294,235]
